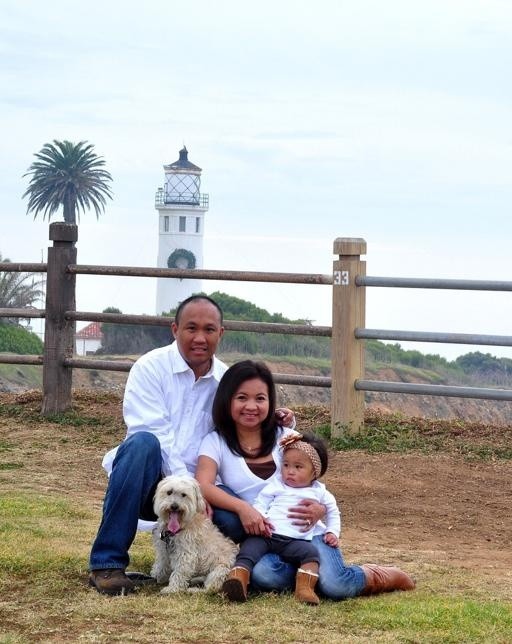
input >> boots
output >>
[296,568,320,604]
[224,568,249,602]
[359,563,415,594]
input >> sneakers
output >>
[89,568,140,594]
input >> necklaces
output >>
[239,439,261,453]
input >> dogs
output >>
[150,474,240,595]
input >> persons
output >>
[193,358,416,603]
[87,295,298,596]
[221,433,343,606]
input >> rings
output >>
[306,519,311,525]
